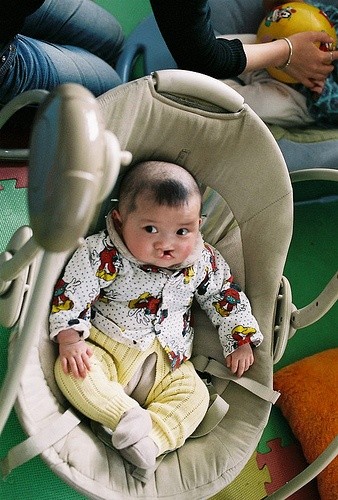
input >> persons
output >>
[47,159,264,470]
[0,0,338,150]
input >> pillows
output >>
[273,347,338,500]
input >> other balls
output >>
[257,2,337,83]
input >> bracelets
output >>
[274,36,292,72]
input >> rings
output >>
[330,52,334,63]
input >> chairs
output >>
[0,69,338,500]
